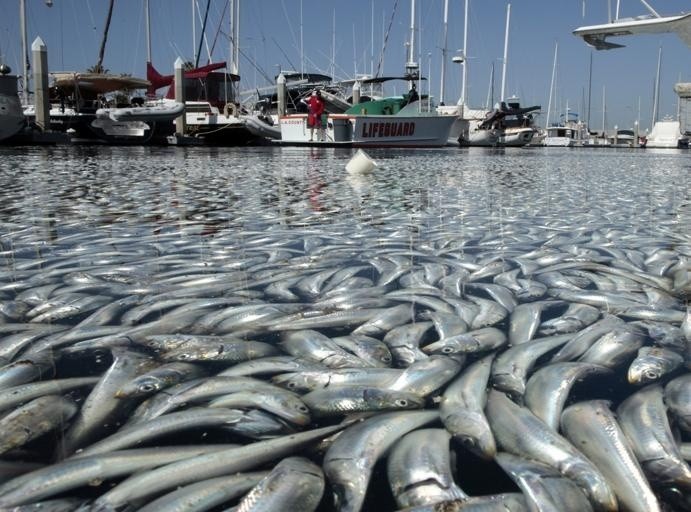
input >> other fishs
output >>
[0,155,691,512]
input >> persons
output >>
[302,89,325,142]
